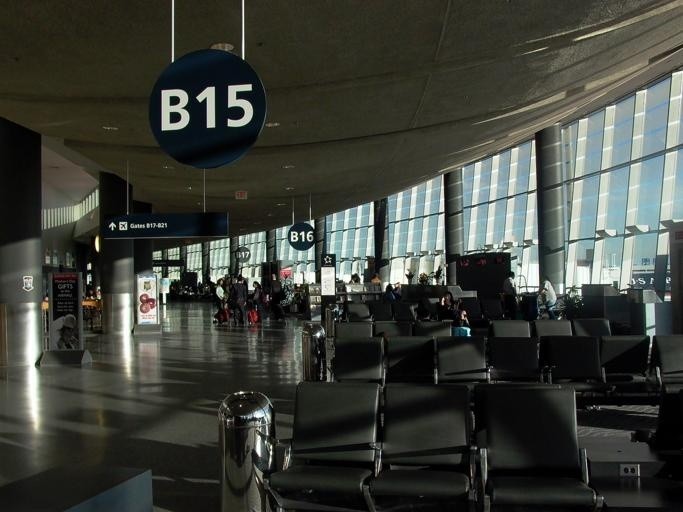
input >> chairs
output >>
[473,382,605,512]
[215,287,681,472]
[262,379,380,511]
[366,383,477,511]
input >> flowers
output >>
[417,273,431,281]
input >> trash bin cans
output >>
[217,390,276,512]
[300,322,328,384]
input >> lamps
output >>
[482,239,538,251]
[295,259,313,265]
[406,249,444,258]
[594,218,679,236]
[339,255,373,262]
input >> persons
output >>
[44,291,47,301]
[170,272,291,327]
[82,281,97,318]
[370,273,383,282]
[393,282,401,299]
[533,274,558,320]
[450,310,470,327]
[348,275,356,283]
[502,271,519,319]
[380,285,395,304]
[353,273,360,283]
[436,291,457,320]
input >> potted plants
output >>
[404,268,415,284]
[430,266,442,284]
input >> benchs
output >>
[2,465,153,511]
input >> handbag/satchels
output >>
[215,295,258,323]
